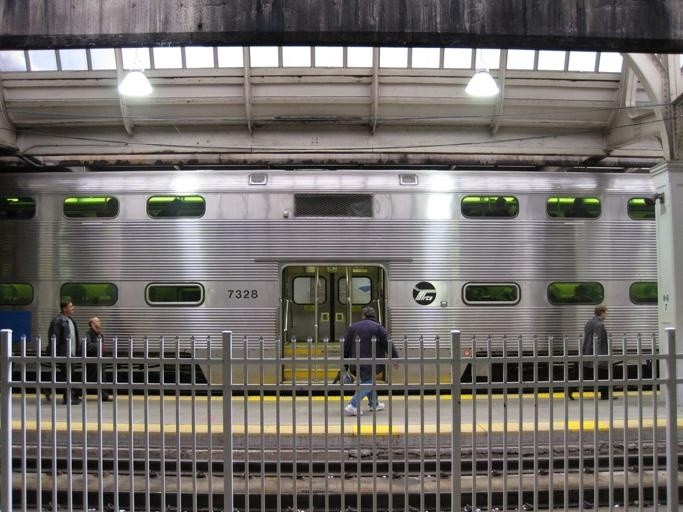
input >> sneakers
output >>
[370,403,385,411]
[345,403,363,415]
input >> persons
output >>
[42,300,81,405]
[76,317,114,402]
[343,306,400,417]
[567,305,618,402]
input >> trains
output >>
[0,169,659,390]
